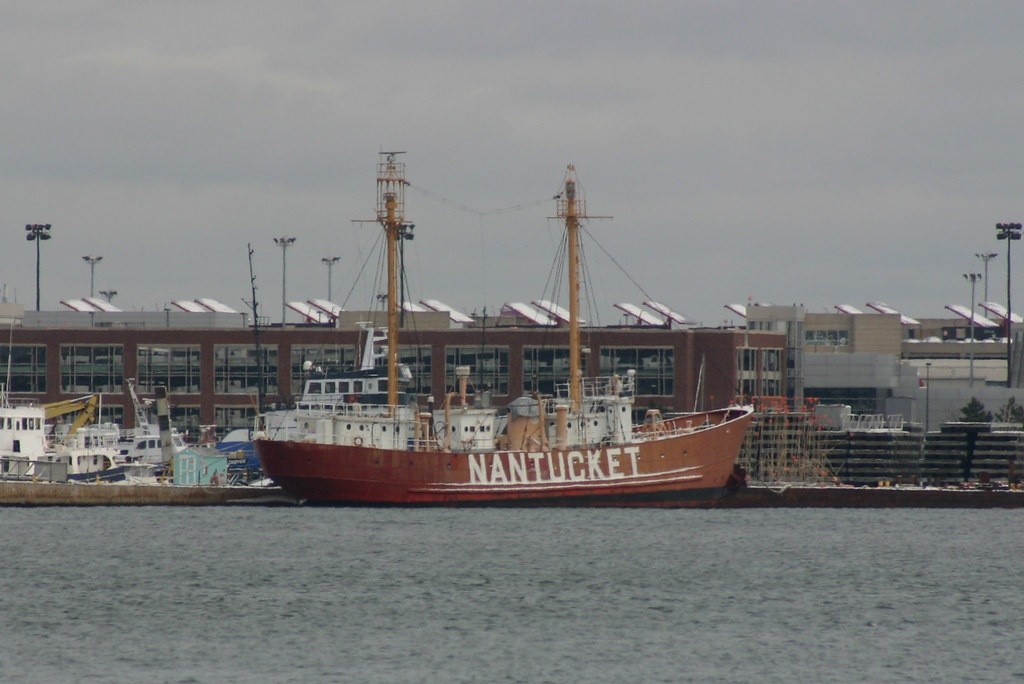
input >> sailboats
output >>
[249,151,754,508]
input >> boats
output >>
[299,321,412,411]
[0,320,275,488]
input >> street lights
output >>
[273,237,296,328]
[926,362,931,431]
[88,312,95,326]
[962,272,983,388]
[164,308,171,327]
[82,256,102,298]
[98,291,117,305]
[322,257,341,302]
[995,220,1022,388]
[240,312,248,328]
[974,252,998,319]
[25,223,51,312]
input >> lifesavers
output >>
[354,437,363,446]
